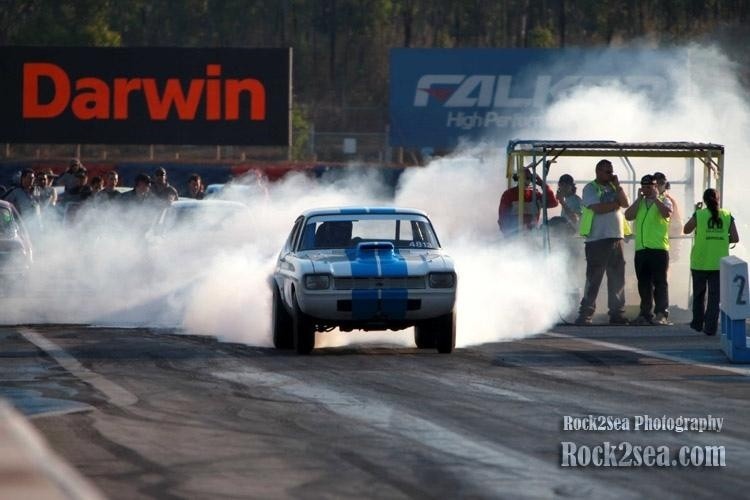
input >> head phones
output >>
[513,167,533,182]
[655,173,670,189]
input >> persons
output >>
[684,188,738,335]
[497,167,558,240]
[1,156,400,218]
[574,159,632,326]
[548,172,583,235]
[624,174,674,329]
[655,172,682,270]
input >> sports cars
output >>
[266,206,458,355]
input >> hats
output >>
[559,174,573,184]
[640,174,657,185]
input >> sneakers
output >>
[575,316,593,325]
[630,315,655,326]
[610,317,630,326]
[651,312,668,325]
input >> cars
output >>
[1,201,34,292]
[144,199,252,273]
